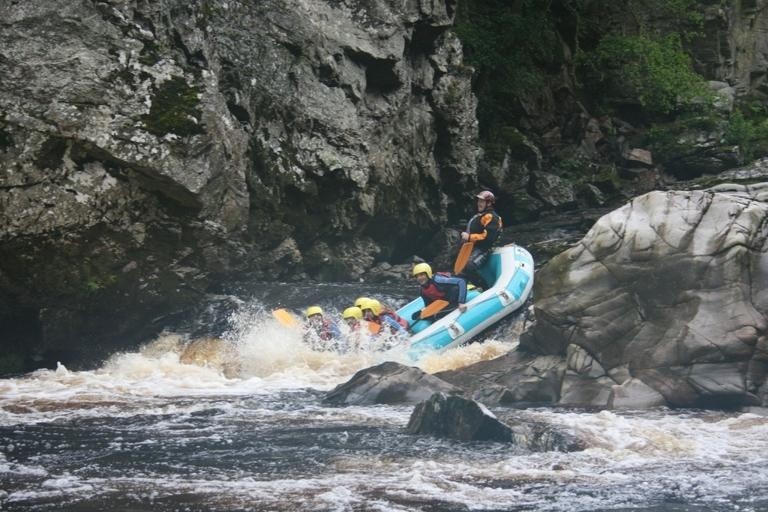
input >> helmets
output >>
[307,306,323,317]
[476,190,495,204]
[343,297,380,321]
[412,263,432,279]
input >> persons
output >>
[449,188,504,294]
[272,297,412,359]
[411,262,468,326]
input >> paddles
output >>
[454,242,474,277]
[406,300,450,332]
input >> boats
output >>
[339,242,536,364]
